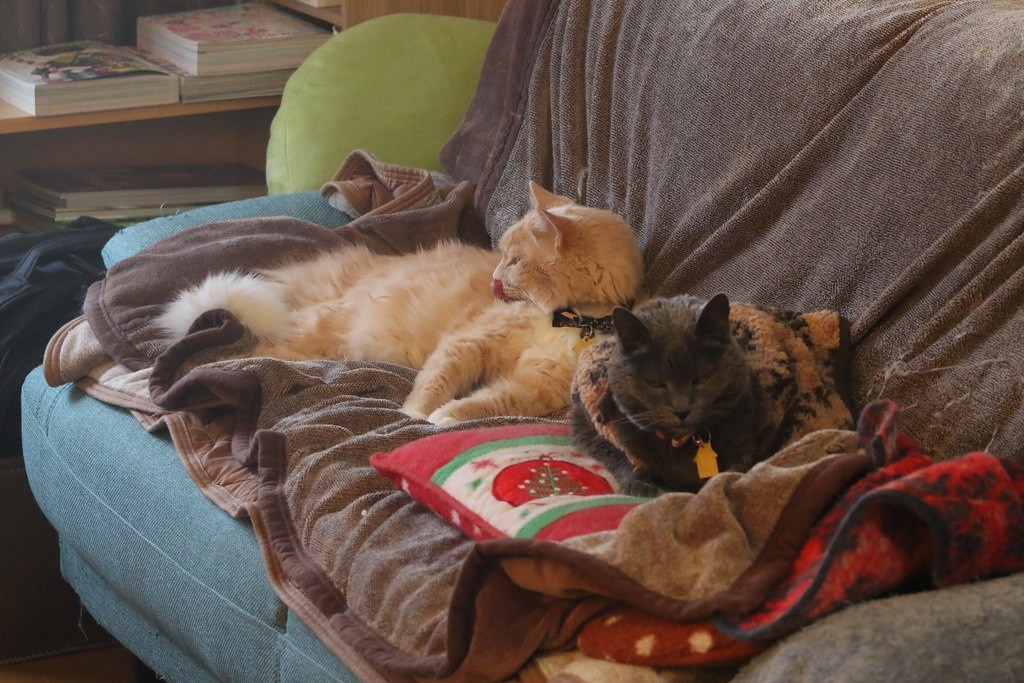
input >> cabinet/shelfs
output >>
[0,0,508,262]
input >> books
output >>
[123,0,341,105]
[0,163,269,234]
[0,39,180,118]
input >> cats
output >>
[564,293,776,498]
[147,180,642,428]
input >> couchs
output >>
[20,1,1024,683]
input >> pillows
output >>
[370,419,656,564]
[264,15,499,203]
